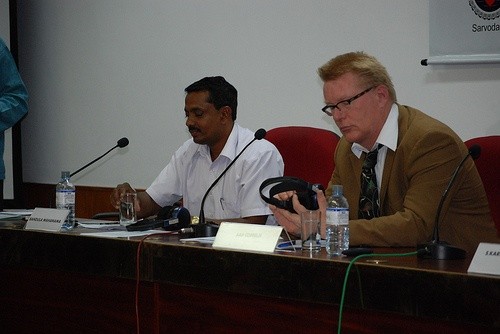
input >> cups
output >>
[119,193,137,226]
[300,210,322,253]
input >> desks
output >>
[0,215,500,334]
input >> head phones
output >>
[126,205,190,232]
[259,176,325,214]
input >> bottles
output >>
[326,185,350,256]
[55,170,76,230]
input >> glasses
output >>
[321,87,373,117]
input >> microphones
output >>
[71,137,129,177]
[417,143,481,259]
[188,128,267,238]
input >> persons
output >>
[269,51,500,254]
[109,75,285,225]
[0,37,29,212]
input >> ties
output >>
[359,144,385,219]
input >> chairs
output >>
[464,135,500,236]
[265,127,340,192]
[52,187,146,218]
